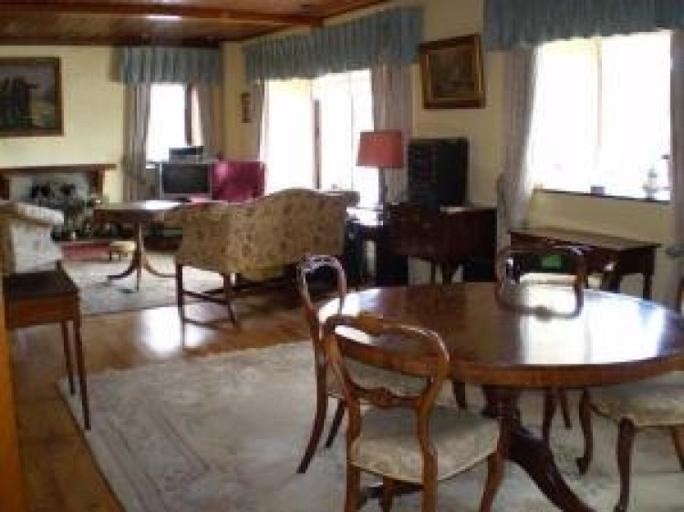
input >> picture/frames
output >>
[0,56,64,137]
[415,31,486,110]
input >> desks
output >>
[507,223,664,301]
[343,196,498,290]
[94,200,184,292]
[3,261,91,432]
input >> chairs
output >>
[187,156,267,205]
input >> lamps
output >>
[356,127,405,212]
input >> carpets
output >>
[54,337,682,509]
[64,249,235,316]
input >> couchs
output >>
[172,187,360,324]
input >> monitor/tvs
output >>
[156,162,213,200]
